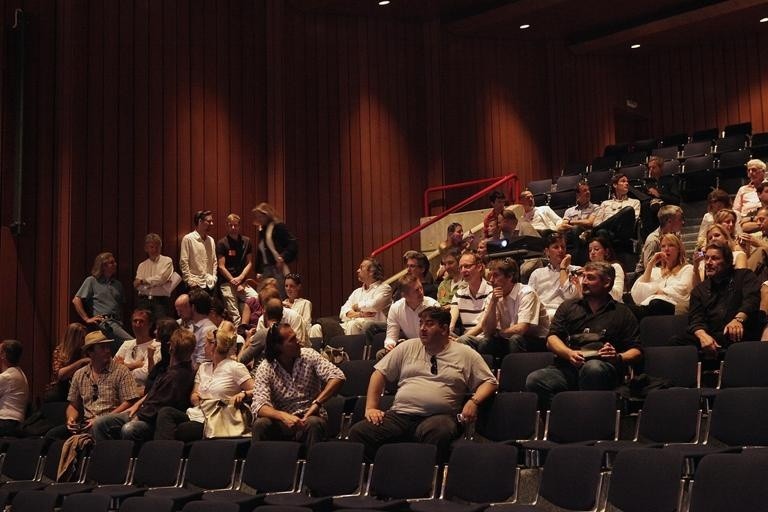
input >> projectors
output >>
[483,235,548,259]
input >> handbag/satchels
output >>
[564,331,611,362]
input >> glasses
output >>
[430,353,438,375]
[92,383,98,401]
[132,346,138,358]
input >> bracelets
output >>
[471,396,480,406]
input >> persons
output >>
[312,256,393,338]
[348,306,499,465]
[0,340,29,438]
[35,202,346,456]
[376,156,767,361]
[524,261,646,424]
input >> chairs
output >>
[529,122,767,206]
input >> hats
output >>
[81,330,115,350]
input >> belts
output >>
[139,295,168,301]
[102,314,122,321]
